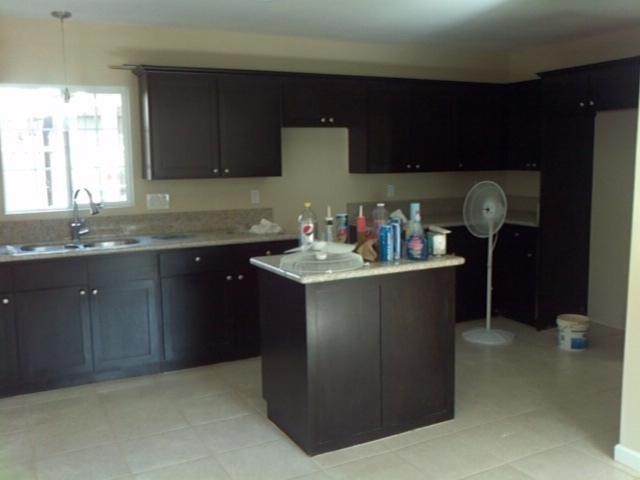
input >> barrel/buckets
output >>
[556,313,593,352]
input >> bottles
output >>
[297,200,401,262]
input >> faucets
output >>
[67,187,100,240]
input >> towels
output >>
[252,219,286,236]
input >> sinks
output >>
[80,237,163,248]
[8,243,82,256]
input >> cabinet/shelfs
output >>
[536,57,639,119]
[255,273,460,456]
[129,67,284,181]
[347,77,539,175]
[279,73,365,128]
[445,224,537,330]
[0,231,303,397]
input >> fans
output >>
[459,181,516,345]
